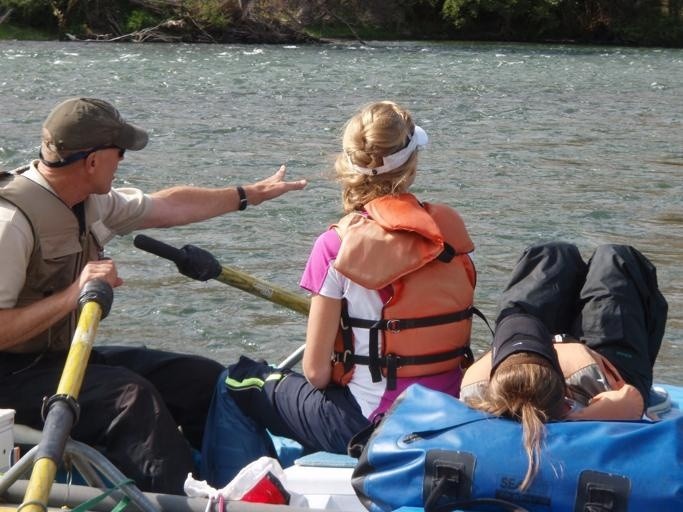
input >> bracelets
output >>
[237,186,247,212]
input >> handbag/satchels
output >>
[350,383,683,512]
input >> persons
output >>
[457,238,670,495]
[0,95,310,495]
[200,98,496,505]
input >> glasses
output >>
[101,145,125,157]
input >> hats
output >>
[488,315,565,404]
[41,99,147,152]
[344,125,428,174]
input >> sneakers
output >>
[645,385,671,414]
